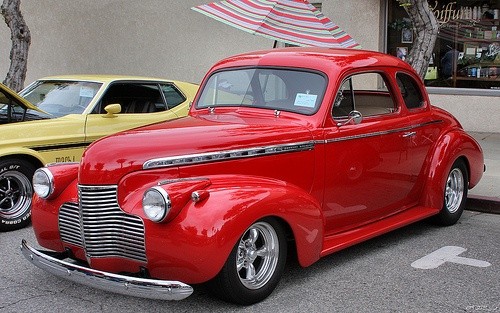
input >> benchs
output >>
[355,95,394,115]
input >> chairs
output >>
[125,100,155,114]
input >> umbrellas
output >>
[190,0,359,49]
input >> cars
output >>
[440,62,500,90]
[1,73,254,233]
[14,45,488,308]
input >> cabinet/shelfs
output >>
[454,19,500,89]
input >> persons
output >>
[439,46,462,75]
